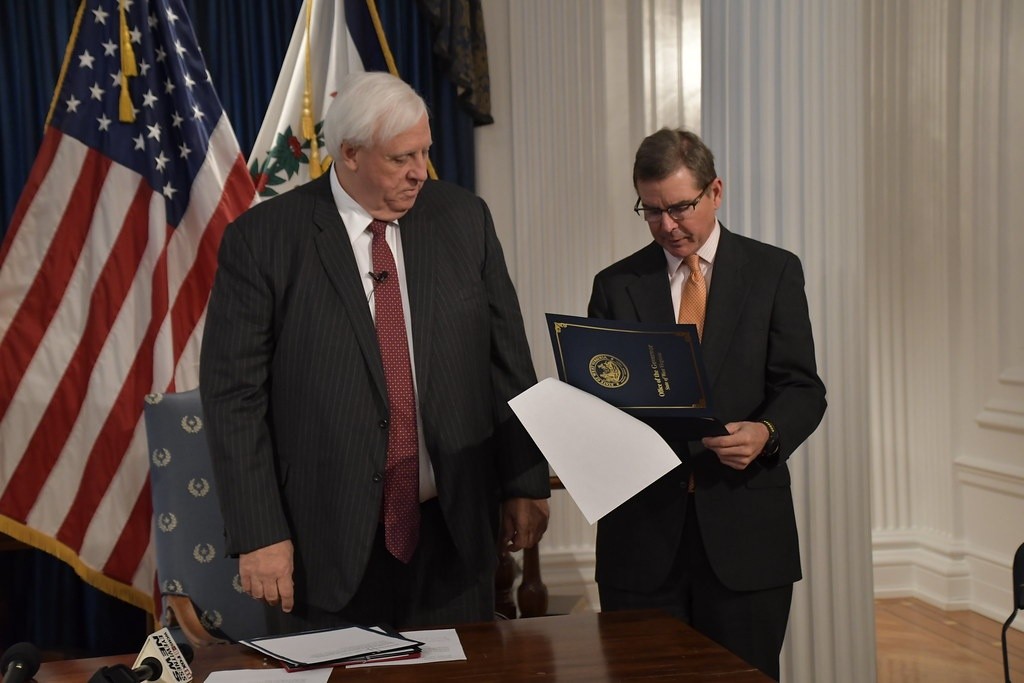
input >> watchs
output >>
[762,418,779,459]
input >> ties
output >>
[366,217,420,565]
[677,254,707,495]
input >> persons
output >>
[199,72,551,636]
[586,127,828,683]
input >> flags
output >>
[247,0,437,203]
[0,0,269,623]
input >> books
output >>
[233,626,467,674]
[545,313,730,465]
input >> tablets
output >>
[277,621,421,674]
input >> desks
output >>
[29,603,785,683]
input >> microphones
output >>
[86,627,195,683]
[0,642,42,683]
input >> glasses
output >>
[634,178,715,222]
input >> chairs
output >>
[146,386,269,648]
